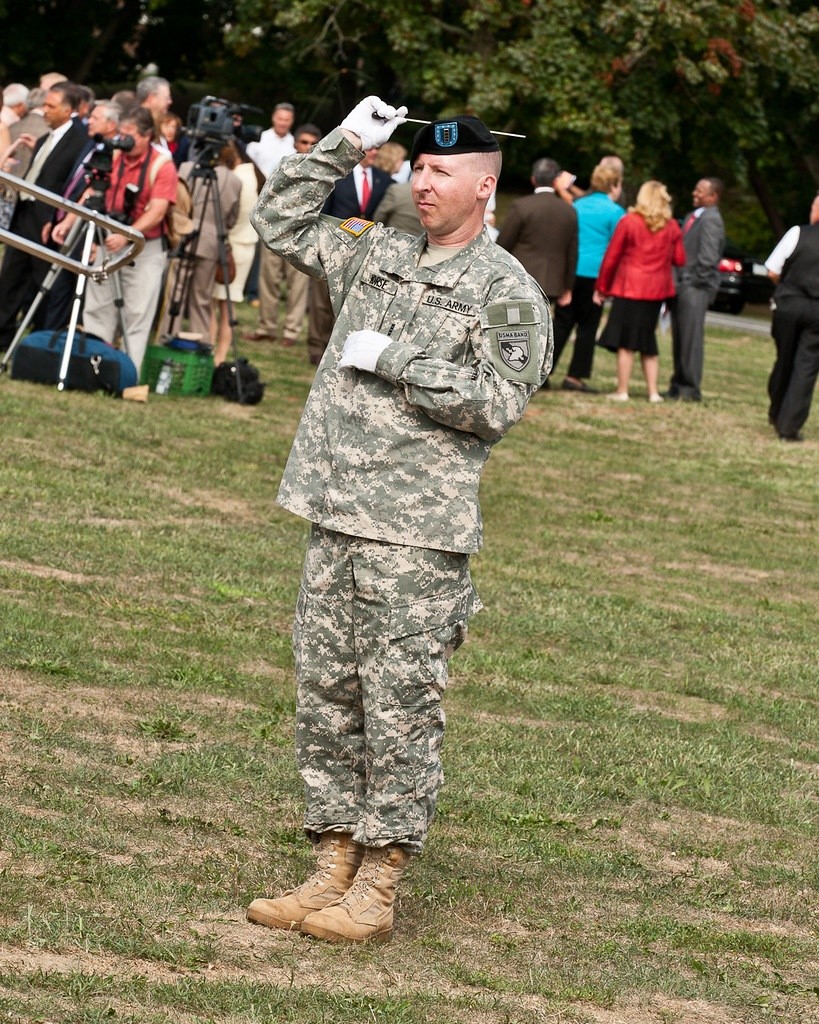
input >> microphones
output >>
[232,104,263,117]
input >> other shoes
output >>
[247,334,277,342]
[279,338,297,346]
[606,392,630,402]
[562,377,586,390]
[651,394,664,403]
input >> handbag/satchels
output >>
[215,237,235,285]
[10,325,137,400]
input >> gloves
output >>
[335,329,393,372]
[339,95,407,152]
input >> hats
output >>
[410,115,500,171]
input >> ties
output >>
[18,133,55,202]
[57,168,84,221]
[362,171,369,213]
[683,214,696,232]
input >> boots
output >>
[246,833,366,930]
[299,844,410,945]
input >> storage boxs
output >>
[143,346,219,396]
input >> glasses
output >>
[298,140,317,146]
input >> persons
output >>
[246,95,553,945]
[592,180,686,404]
[495,159,578,315]
[2,71,424,368]
[50,107,176,387]
[546,157,635,392]
[659,178,723,403]
[764,196,819,441]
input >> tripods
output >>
[164,135,246,410]
[0,170,132,393]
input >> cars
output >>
[710,253,747,317]
